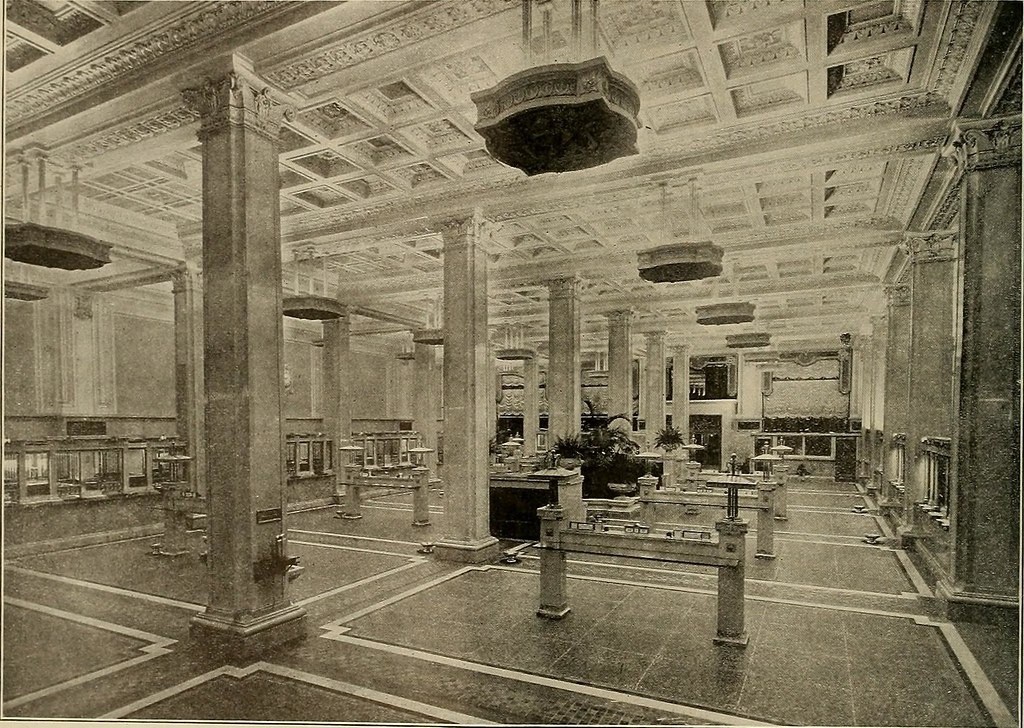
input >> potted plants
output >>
[554,433,580,468]
[607,450,632,494]
[653,425,684,452]
